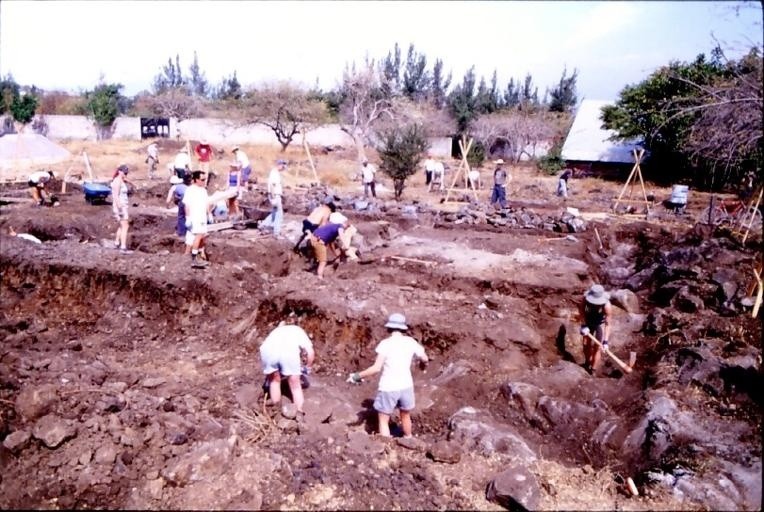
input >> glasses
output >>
[200,178,207,181]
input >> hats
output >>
[118,165,129,175]
[324,195,334,204]
[277,160,286,165]
[49,169,58,180]
[584,285,610,306]
[492,158,506,164]
[384,313,408,330]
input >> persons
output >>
[258,311,315,414]
[423,152,445,193]
[579,285,613,375]
[555,168,572,200]
[111,139,287,267]
[293,201,352,279]
[361,157,378,196]
[490,159,509,204]
[463,171,481,188]
[347,312,430,439]
[28,170,54,207]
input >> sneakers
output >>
[191,252,208,267]
[114,244,133,254]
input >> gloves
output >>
[581,327,590,335]
[602,341,608,352]
